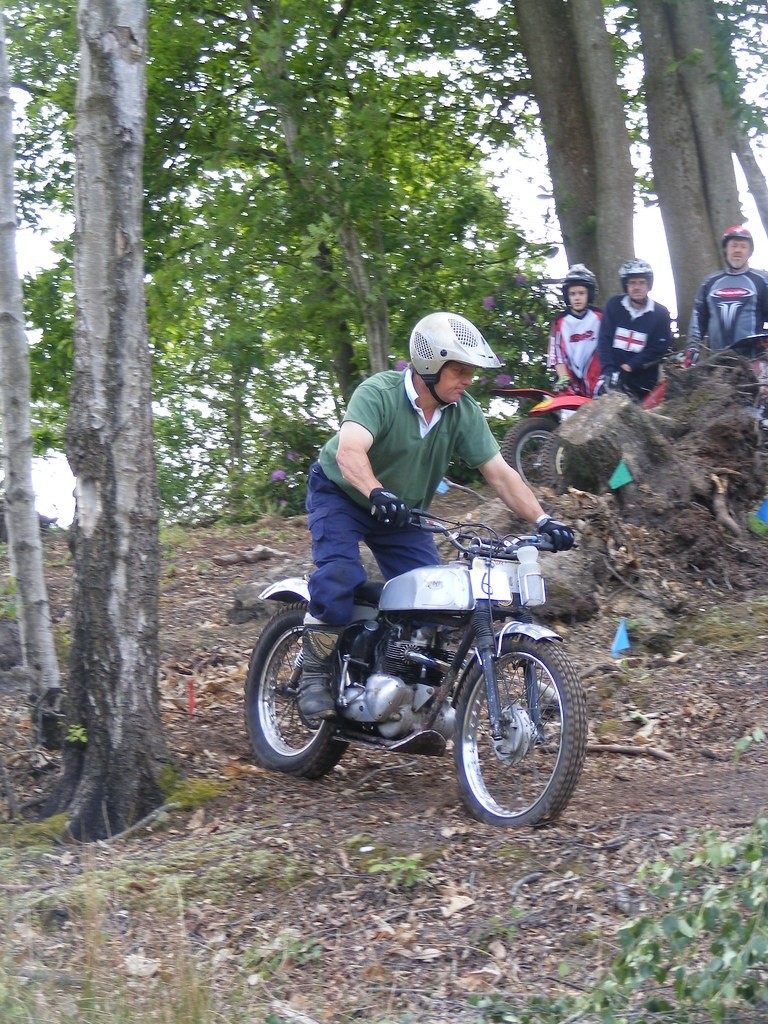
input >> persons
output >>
[546,226,768,427]
[286,311,576,724]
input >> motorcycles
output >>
[489,334,768,488]
[244,506,589,828]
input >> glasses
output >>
[443,364,476,377]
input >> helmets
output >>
[721,225,753,256]
[617,258,653,292]
[410,312,501,386]
[562,264,598,305]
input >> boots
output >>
[410,627,452,651]
[295,612,346,728]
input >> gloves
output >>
[598,366,631,387]
[552,376,580,396]
[684,348,700,367]
[535,515,575,553]
[368,487,412,529]
